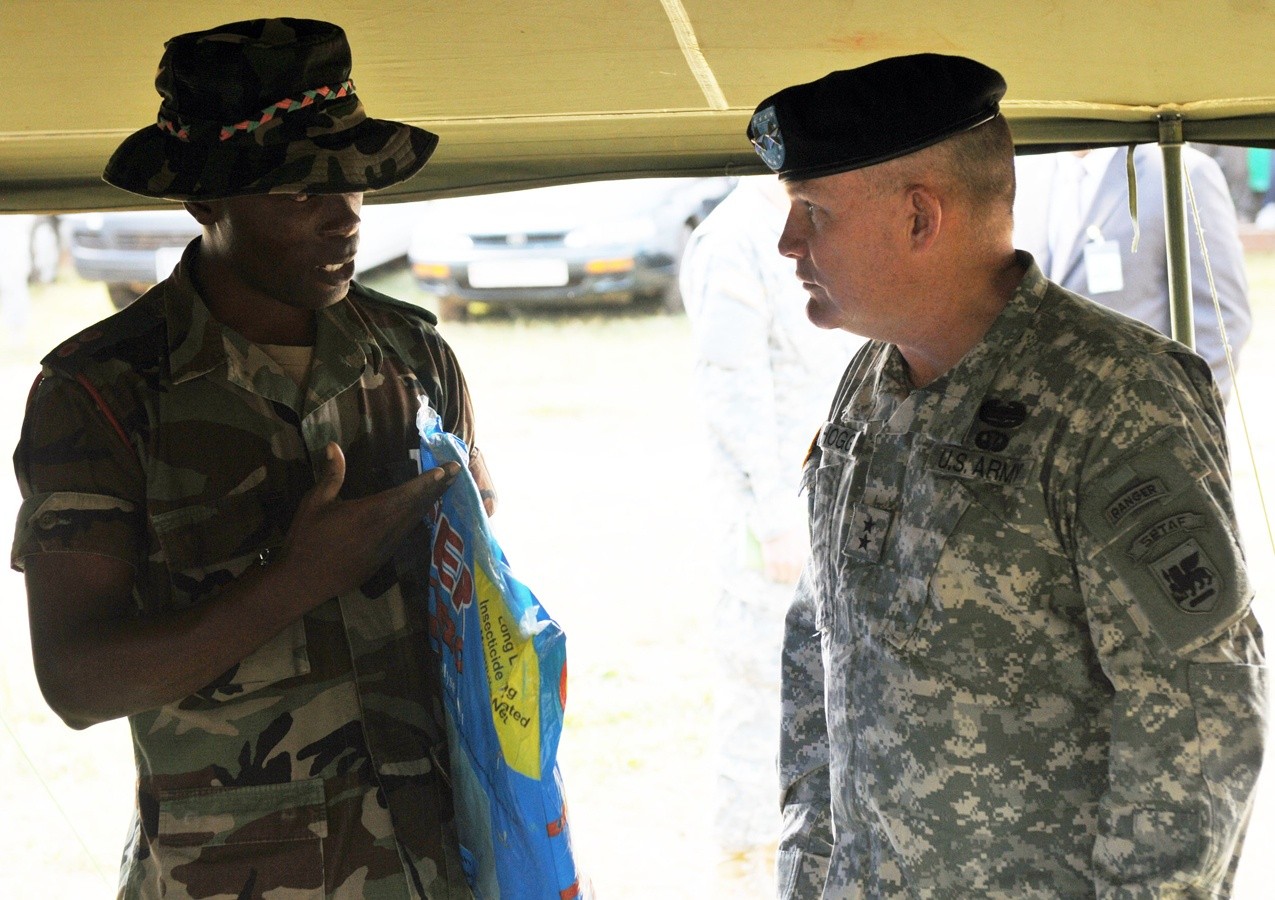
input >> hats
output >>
[99,15,439,200]
[744,54,1012,176]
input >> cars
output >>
[1,207,81,294]
[73,200,408,313]
[401,171,739,328]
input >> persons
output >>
[1014,141,1254,409]
[680,167,871,886]
[9,18,495,900]
[745,54,1269,900]
